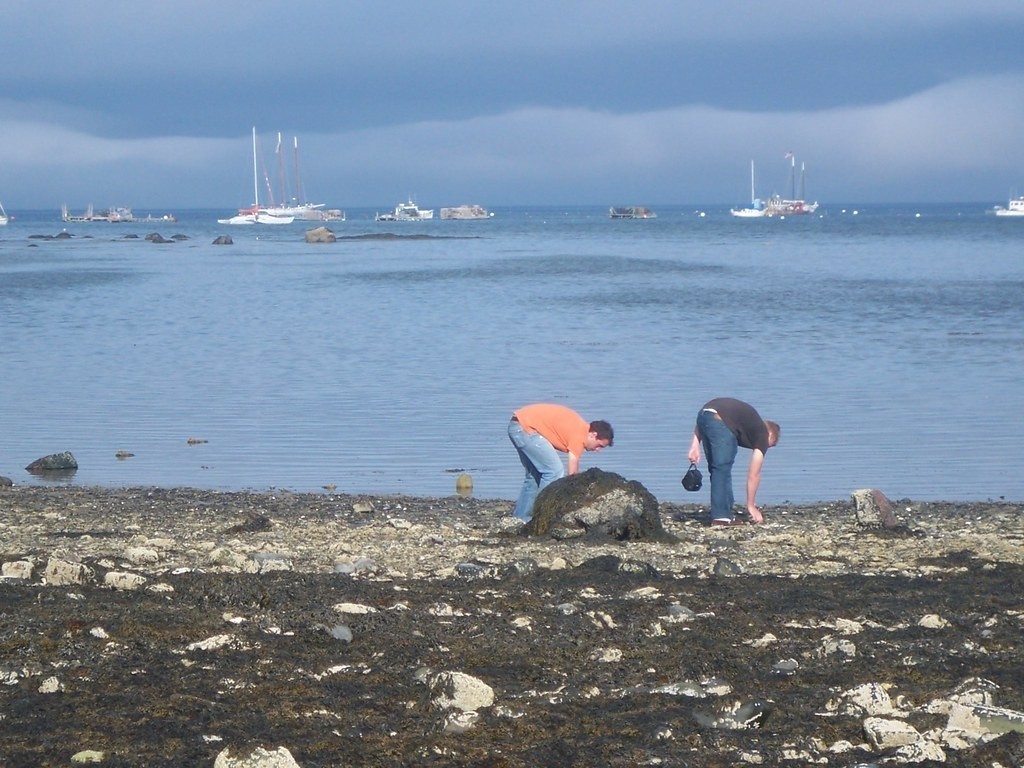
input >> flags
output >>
[784,150,793,158]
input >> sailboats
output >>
[0,203,8,226]
[237,132,345,222]
[732,160,765,210]
[763,152,819,215]
[217,127,296,225]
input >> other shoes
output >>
[711,518,744,526]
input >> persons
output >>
[688,398,780,527]
[507,403,614,525]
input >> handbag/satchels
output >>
[681,463,703,491]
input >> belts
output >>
[511,416,519,421]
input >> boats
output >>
[62,203,176,223]
[984,195,1024,217]
[608,207,658,219]
[730,208,767,217]
[374,192,433,222]
[440,205,489,220]
